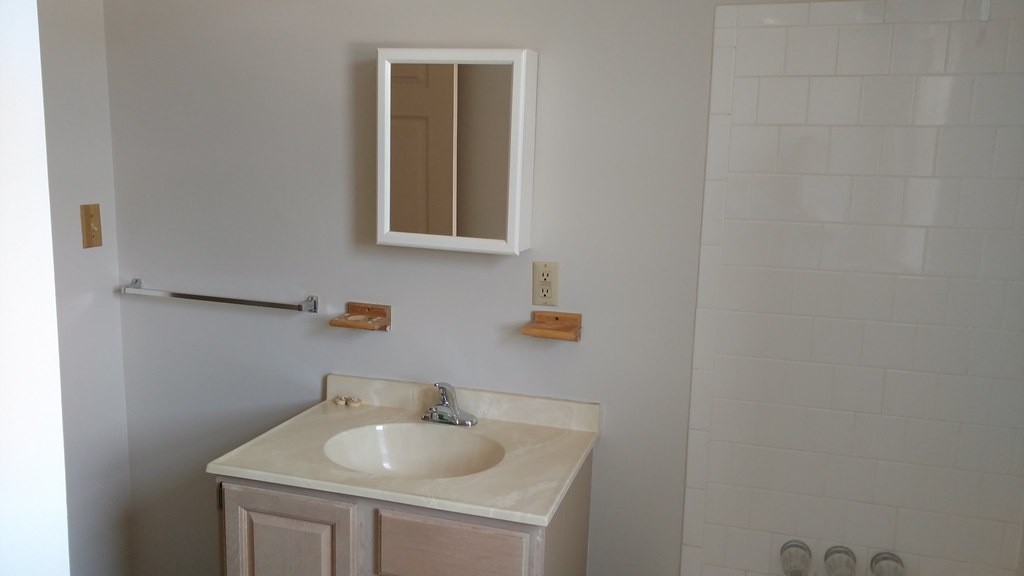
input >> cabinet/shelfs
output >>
[217,476,540,576]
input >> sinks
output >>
[323,422,506,480]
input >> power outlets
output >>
[532,260,559,306]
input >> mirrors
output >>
[374,46,535,256]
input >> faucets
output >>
[418,381,477,426]
